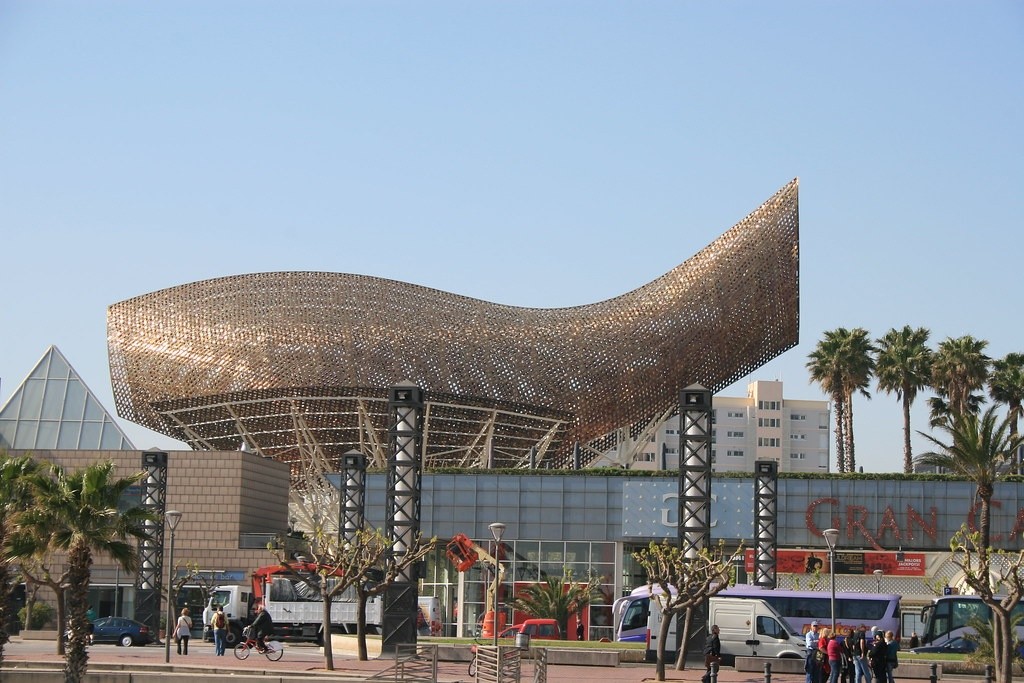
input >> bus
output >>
[612,580,902,644]
[919,593,1024,659]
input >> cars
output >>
[909,635,978,654]
[62,616,157,648]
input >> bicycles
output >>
[467,636,519,679]
[233,628,284,662]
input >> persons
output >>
[211,604,230,656]
[805,621,899,683]
[1016,640,1024,671]
[86,605,97,644]
[909,632,918,648]
[251,605,273,654]
[700,624,722,683]
[417,596,458,636]
[575,619,584,641]
[172,607,192,655]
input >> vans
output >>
[644,595,808,666]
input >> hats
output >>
[811,621,819,626]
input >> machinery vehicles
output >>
[443,533,615,643]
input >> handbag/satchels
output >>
[705,654,719,669]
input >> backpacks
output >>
[215,612,225,628]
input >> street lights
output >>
[822,528,841,633]
[113,558,122,617]
[164,511,182,663]
[873,569,884,593]
[488,523,507,677]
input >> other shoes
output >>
[259,648,268,654]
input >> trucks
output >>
[202,572,382,646]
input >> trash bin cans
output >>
[515,631,531,651]
